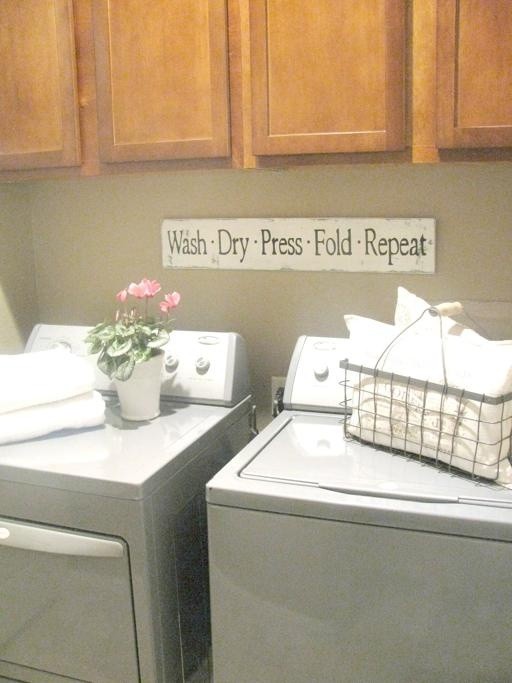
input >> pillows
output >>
[342,286,512,489]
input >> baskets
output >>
[337,301,512,481]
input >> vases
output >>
[106,353,164,423]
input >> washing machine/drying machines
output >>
[0,321,273,682]
[201,334,510,683]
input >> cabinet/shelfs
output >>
[0,1,246,181]
[248,1,511,173]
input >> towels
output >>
[0,347,108,448]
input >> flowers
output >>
[86,276,180,381]
